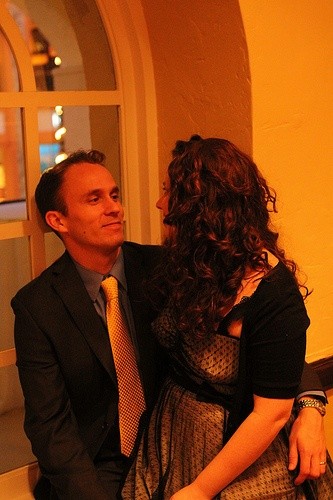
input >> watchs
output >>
[295,399,326,417]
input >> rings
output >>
[320,461,326,465]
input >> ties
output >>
[99,275,147,456]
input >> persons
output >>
[119,133,312,500]
[9,145,329,500]
